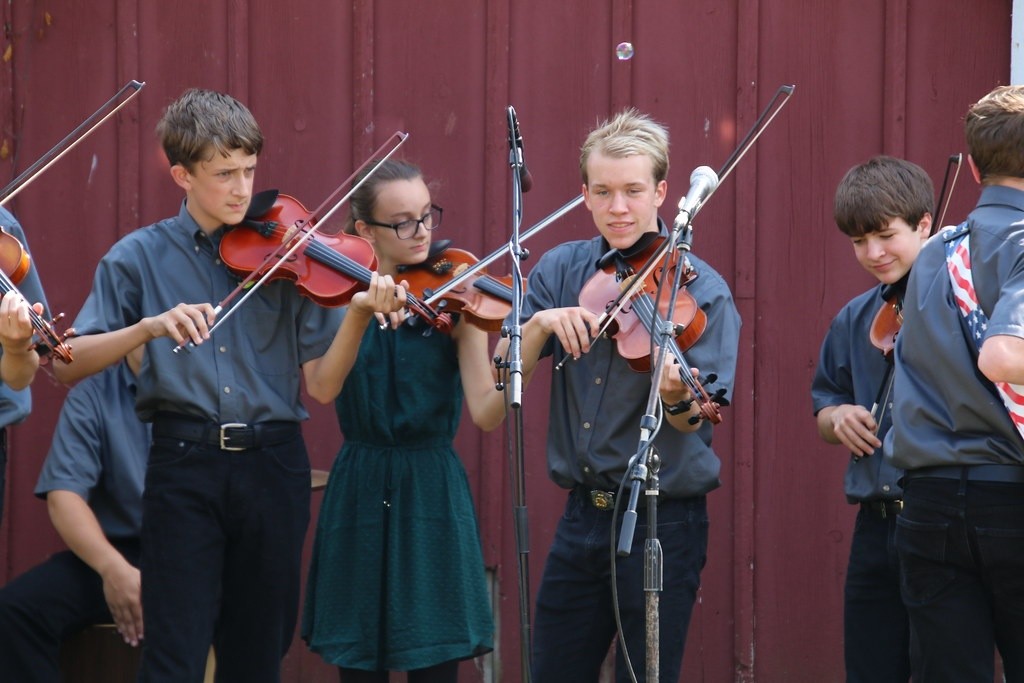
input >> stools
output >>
[63,624,217,683]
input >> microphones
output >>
[506,105,534,193]
[673,166,718,232]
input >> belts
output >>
[576,483,668,512]
[151,416,300,452]
[907,464,1024,483]
[861,500,904,514]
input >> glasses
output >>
[354,203,443,240]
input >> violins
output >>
[387,238,533,331]
[215,185,457,339]
[577,233,729,428]
[0,225,81,367]
[869,274,910,353]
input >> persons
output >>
[0,200,55,515]
[48,87,410,683]
[0,341,229,683]
[809,154,964,682]
[884,80,1024,682]
[300,159,539,683]
[492,108,742,683]
